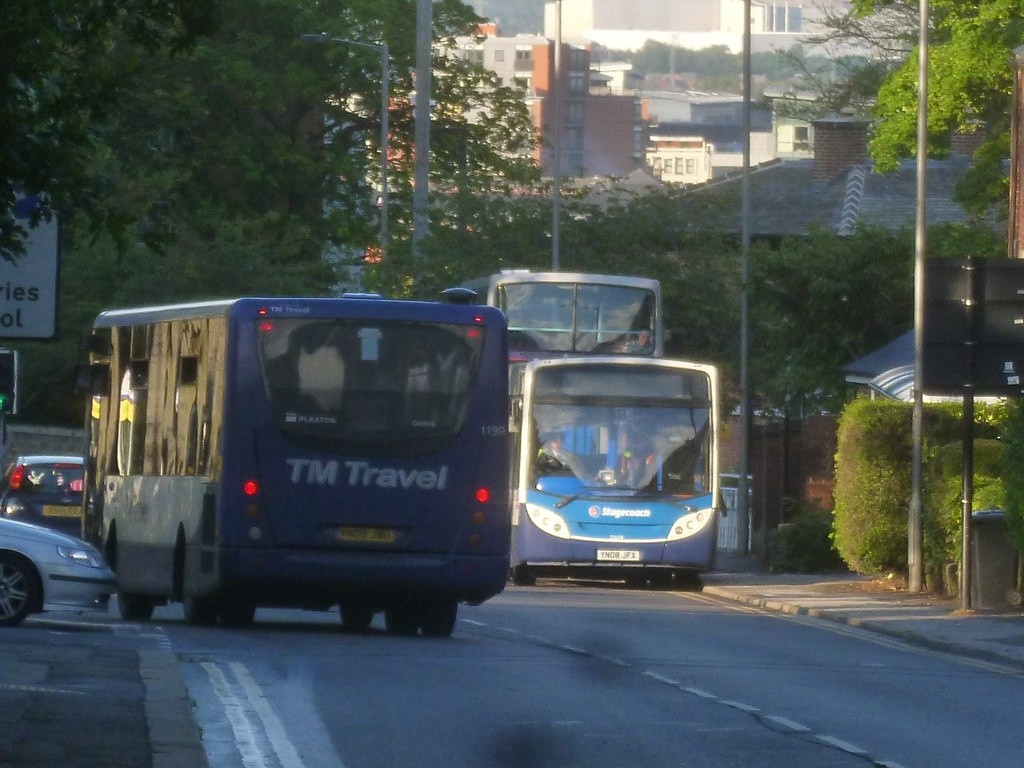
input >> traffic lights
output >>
[0,348,18,415]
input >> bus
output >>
[460,269,664,376]
[509,355,729,590]
[74,287,511,635]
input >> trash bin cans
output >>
[718,473,752,550]
[970,509,1024,612]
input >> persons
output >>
[627,331,650,352]
[537,438,564,460]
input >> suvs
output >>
[0,455,85,536]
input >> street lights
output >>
[302,32,389,282]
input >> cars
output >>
[0,518,116,628]
